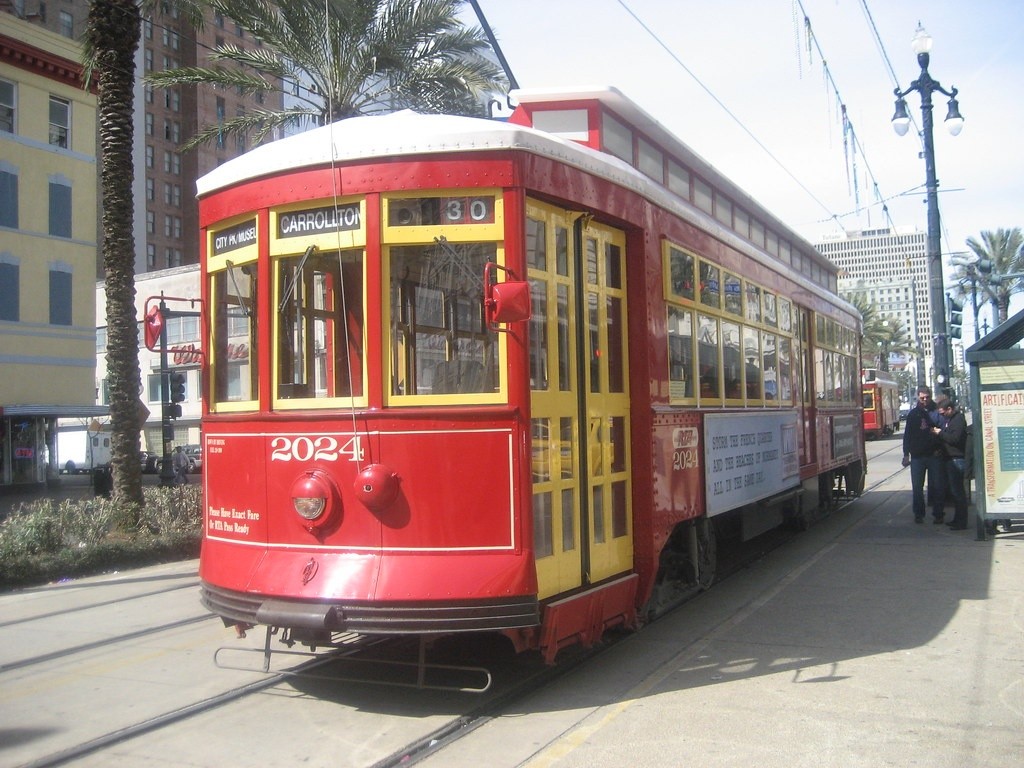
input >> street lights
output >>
[891,20,967,508]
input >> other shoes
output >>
[915,514,924,523]
[933,515,943,523]
[946,521,957,526]
[950,525,966,530]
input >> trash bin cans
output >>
[92,466,113,499]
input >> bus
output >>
[860,368,900,441]
[141,84,870,698]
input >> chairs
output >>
[431,358,485,396]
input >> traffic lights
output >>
[170,373,185,405]
[944,292,963,339]
[172,405,182,418]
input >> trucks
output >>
[45,431,111,475]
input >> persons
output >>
[699,351,776,400]
[930,399,968,531]
[902,385,944,525]
[174,446,190,485]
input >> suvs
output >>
[156,444,203,474]
[141,451,159,474]
